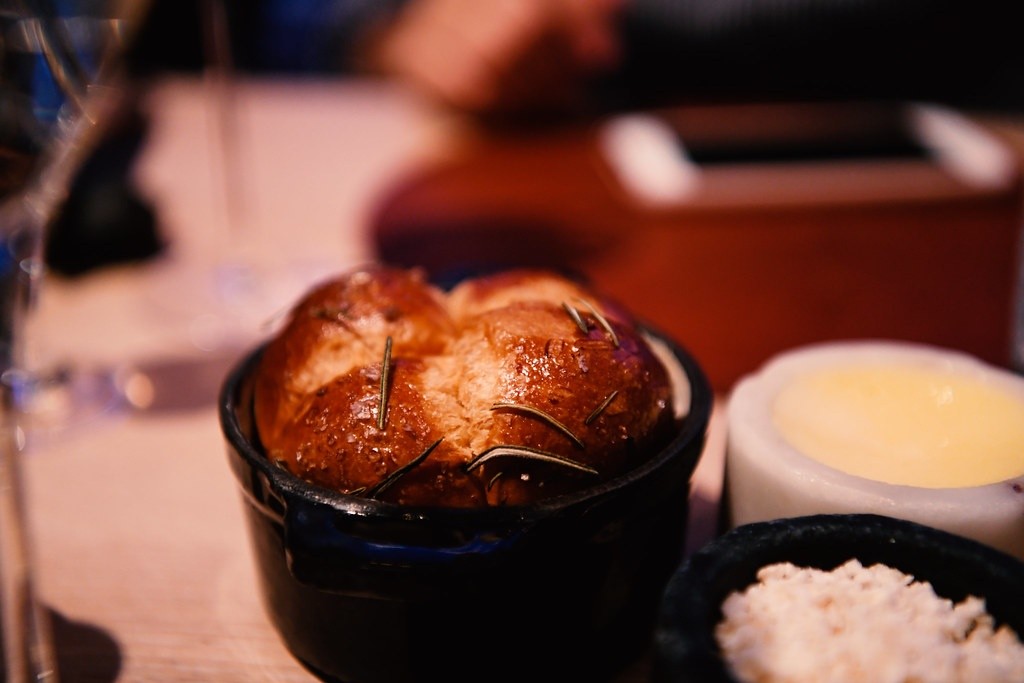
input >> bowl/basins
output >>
[726,340,1024,556]
[216,269,714,683]
[654,510,1024,683]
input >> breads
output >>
[247,257,675,510]
